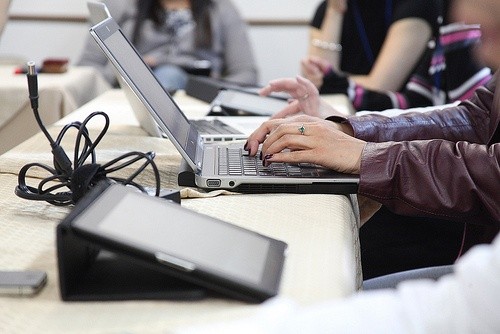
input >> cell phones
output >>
[0,271,47,295]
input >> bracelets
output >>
[312,38,342,53]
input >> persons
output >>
[300,0,494,280]
[243,68,500,292]
[76,0,259,95]
[174,0,500,334]
[309,0,448,93]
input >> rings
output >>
[299,126,305,135]
[302,94,308,100]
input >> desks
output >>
[0,64,114,157]
[0,89,363,334]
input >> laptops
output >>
[85,0,360,195]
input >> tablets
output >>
[71,184,287,297]
[217,88,289,117]
[194,76,264,91]
